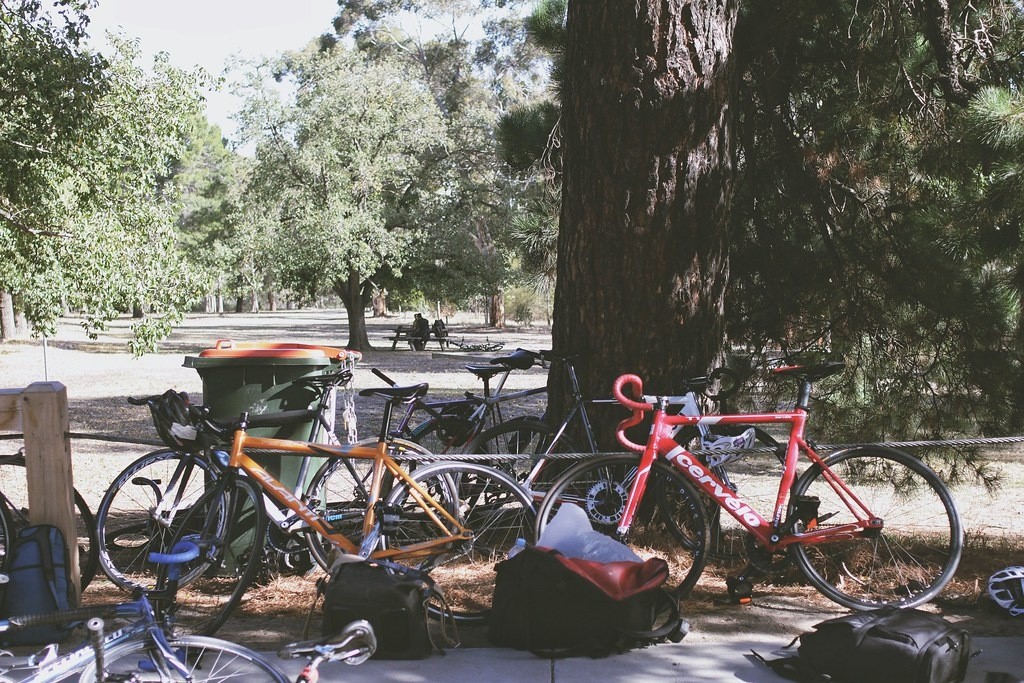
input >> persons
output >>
[410,313,430,351]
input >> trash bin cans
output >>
[182,339,363,577]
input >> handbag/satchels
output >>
[300,553,461,660]
[750,596,972,683]
[491,503,681,659]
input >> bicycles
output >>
[401,342,806,563]
[95,370,461,601]
[450,336,507,352]
[536,360,967,616]
[149,382,543,652]
[0,540,382,683]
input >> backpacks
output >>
[0,522,85,645]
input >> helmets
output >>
[148,389,203,452]
[989,565,1024,616]
[701,424,755,468]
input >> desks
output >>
[391,328,452,352]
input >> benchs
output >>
[384,336,457,341]
